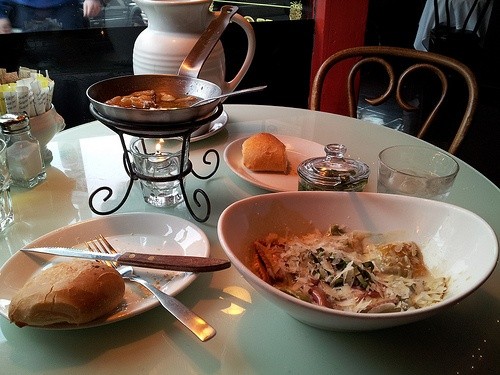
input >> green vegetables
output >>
[278,224,375,306]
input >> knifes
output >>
[19,246,232,273]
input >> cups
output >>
[29,102,61,165]
[375,144,460,202]
[0,140,15,235]
[297,143,370,193]
[130,137,191,209]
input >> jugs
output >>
[132,0,256,139]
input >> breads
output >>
[242,132,289,172]
[7,259,125,326]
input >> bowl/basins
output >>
[217,191,500,332]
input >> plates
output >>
[224,134,328,191]
[177,105,229,143]
[0,212,210,330]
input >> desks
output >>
[0,104,500,375]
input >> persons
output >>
[0,0,102,34]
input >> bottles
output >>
[0,111,46,189]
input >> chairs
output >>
[310,46,479,157]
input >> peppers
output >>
[309,288,326,306]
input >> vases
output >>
[132,0,256,138]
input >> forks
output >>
[84,233,217,342]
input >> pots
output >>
[86,5,239,125]
